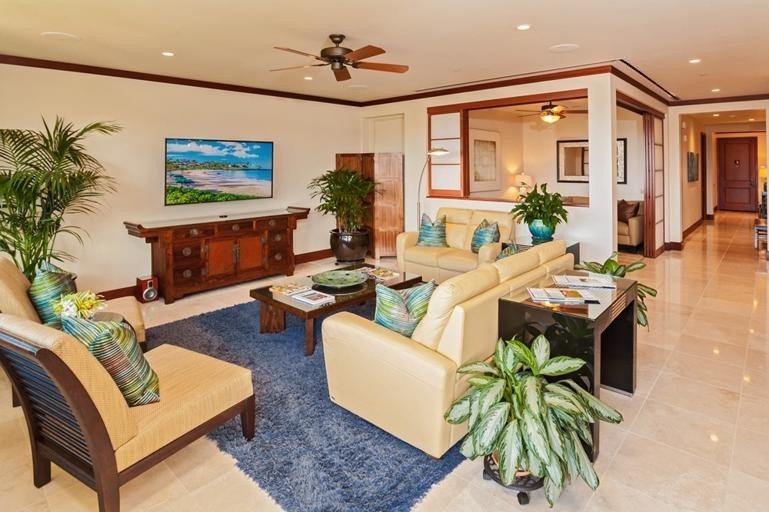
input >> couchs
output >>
[563,194,645,254]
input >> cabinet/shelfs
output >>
[150,209,297,306]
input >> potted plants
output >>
[306,163,384,264]
[444,330,624,509]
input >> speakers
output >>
[136,276,159,303]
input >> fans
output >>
[514,101,589,117]
[269,34,409,82]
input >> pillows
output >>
[617,198,640,224]
[508,181,568,240]
[29,259,77,324]
[62,299,160,404]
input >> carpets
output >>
[135,269,477,512]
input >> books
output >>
[534,271,616,303]
[268,282,335,313]
[360,266,398,282]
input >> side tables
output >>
[502,237,580,267]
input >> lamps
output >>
[513,172,532,203]
[416,148,449,232]
[540,105,560,123]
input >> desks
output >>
[497,268,639,464]
[753,218,767,248]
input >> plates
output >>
[312,281,368,296]
[311,270,370,287]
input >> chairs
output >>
[0,256,146,408]
[0,311,254,512]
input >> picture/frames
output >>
[557,137,627,184]
[468,128,501,192]
